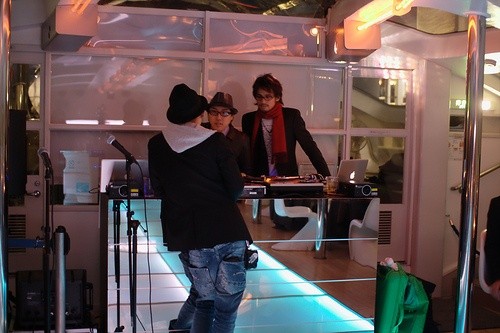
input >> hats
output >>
[205,91,238,116]
[166,83,208,126]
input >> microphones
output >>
[107,136,131,162]
[38,147,51,166]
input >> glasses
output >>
[256,94,275,101]
[209,110,231,117]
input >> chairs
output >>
[272,199,383,269]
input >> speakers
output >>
[350,184,372,198]
[108,180,142,199]
[16,268,88,328]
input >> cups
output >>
[326,176,339,195]
[143,177,155,198]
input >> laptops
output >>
[337,158,368,188]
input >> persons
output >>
[201,92,252,178]
[242,74,331,178]
[148,83,253,333]
